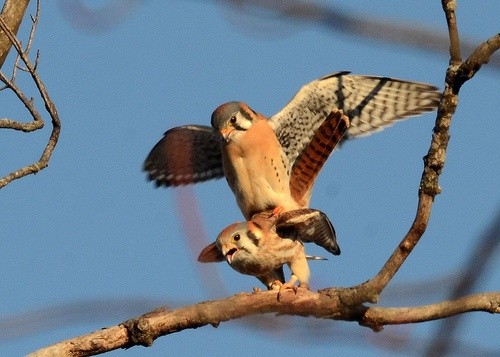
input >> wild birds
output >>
[141,69,445,220]
[196,208,341,302]
